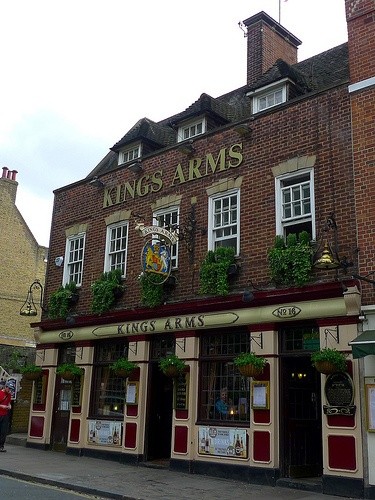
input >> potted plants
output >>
[20,362,43,380]
[310,347,348,375]
[90,269,126,315]
[157,355,186,378]
[109,358,135,378]
[233,351,266,377]
[138,270,177,309]
[55,364,82,380]
[50,282,79,320]
[197,246,238,296]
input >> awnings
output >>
[348,330,375,359]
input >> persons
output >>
[0,382,14,452]
[216,388,237,419]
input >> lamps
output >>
[178,144,196,155]
[309,216,342,270]
[89,179,104,188]
[232,124,252,135]
[127,163,142,174]
[19,281,48,317]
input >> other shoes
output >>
[0,447,6,452]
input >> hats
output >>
[5,382,15,390]
